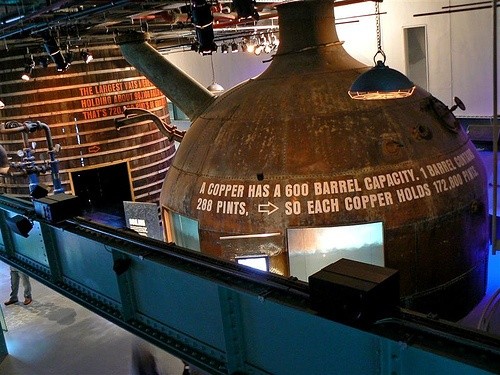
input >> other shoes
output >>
[24,299,32,305]
[4,300,19,306]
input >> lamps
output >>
[180,0,278,56]
[14,21,93,81]
[207,56,225,93]
[348,0,416,100]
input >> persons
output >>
[4,264,32,305]
